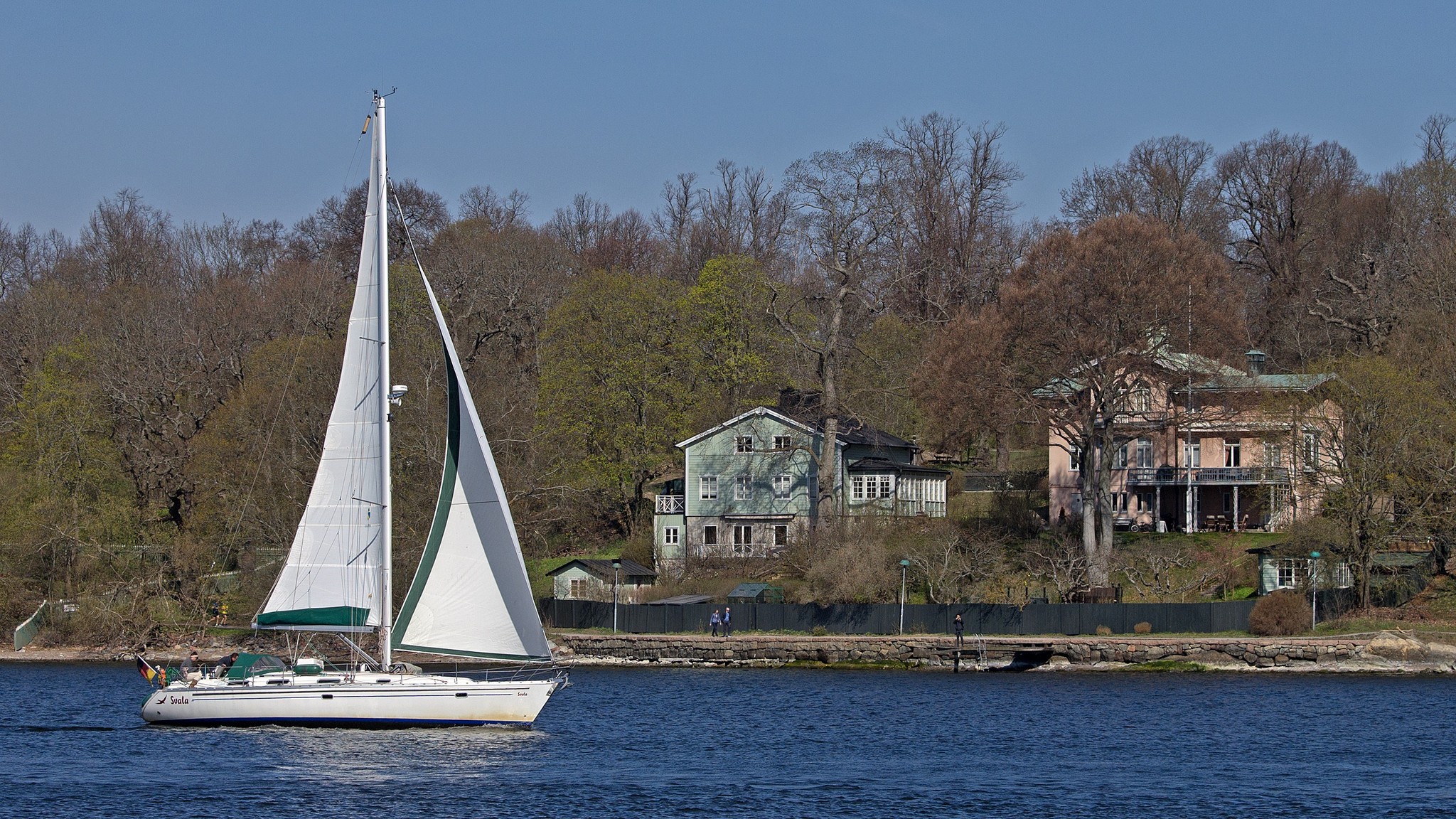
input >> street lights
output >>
[1310,551,1321,631]
[613,562,622,633]
[899,559,910,636]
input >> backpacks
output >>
[213,608,218,617]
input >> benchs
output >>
[1113,517,1133,532]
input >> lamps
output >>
[266,679,289,684]
[227,681,249,685]
[317,678,340,683]
[376,679,391,683]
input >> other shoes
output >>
[216,624,218,626]
[223,625,227,627]
[219,624,223,627]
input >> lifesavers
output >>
[159,668,167,686]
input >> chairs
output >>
[1198,515,1248,534]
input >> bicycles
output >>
[1131,516,1150,532]
[1149,516,1158,532]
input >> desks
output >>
[1215,519,1232,532]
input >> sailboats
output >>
[141,84,574,729]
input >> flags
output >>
[137,656,157,687]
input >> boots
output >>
[189,679,198,689]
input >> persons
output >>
[709,609,722,638]
[722,607,732,637]
[179,651,202,689]
[208,652,238,680]
[155,665,166,689]
[953,614,965,647]
